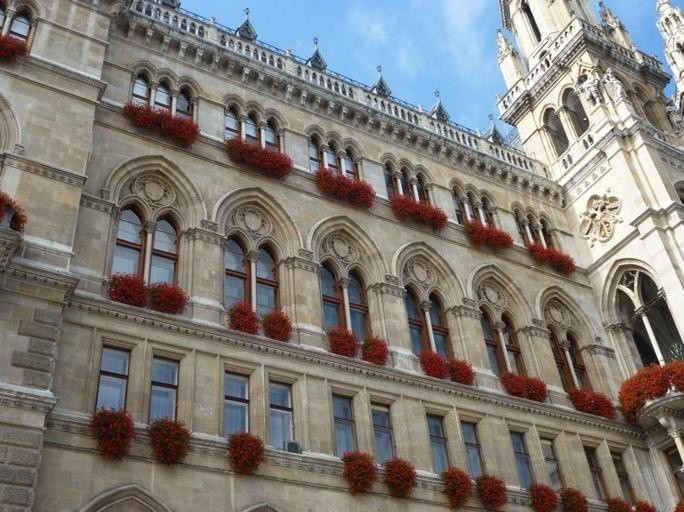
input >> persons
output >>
[664,99,683,133]
[577,75,601,105]
[604,68,627,100]
[598,2,627,32]
